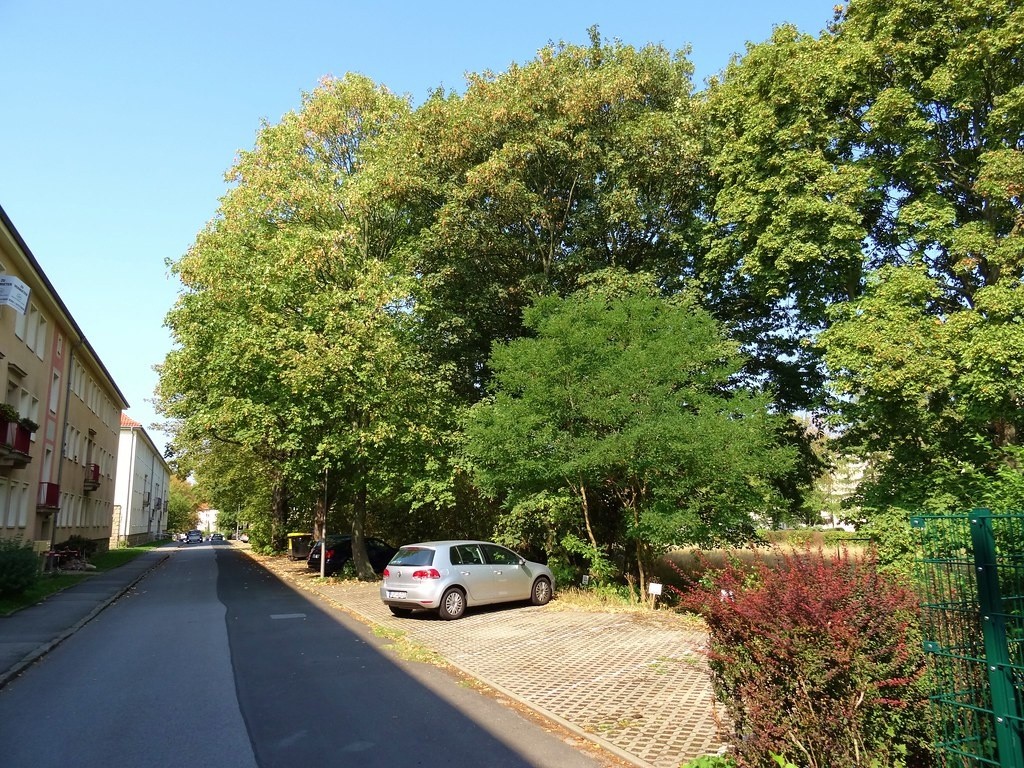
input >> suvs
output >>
[307,535,401,575]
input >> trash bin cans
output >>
[287,532,313,561]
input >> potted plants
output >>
[21,417,40,433]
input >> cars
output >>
[241,532,249,544]
[380,539,557,621]
[185,529,204,544]
[212,533,223,540]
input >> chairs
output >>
[463,551,474,564]
